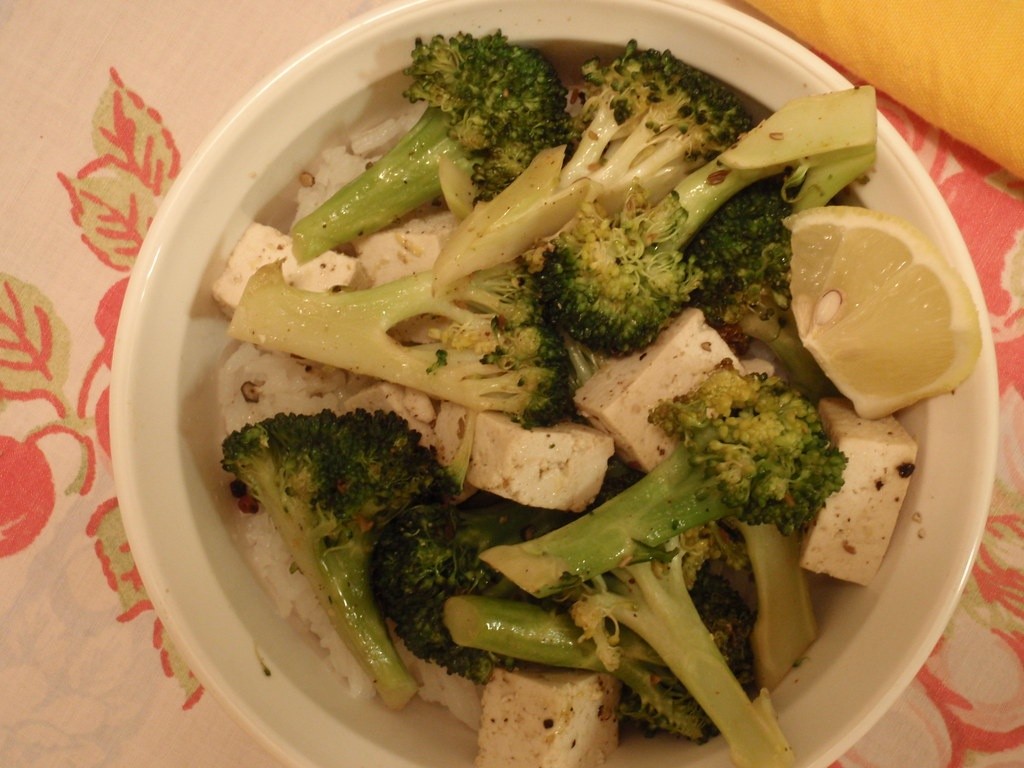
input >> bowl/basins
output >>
[109,0,1000,767]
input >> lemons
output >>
[781,205,982,424]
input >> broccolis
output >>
[216,23,883,767]
